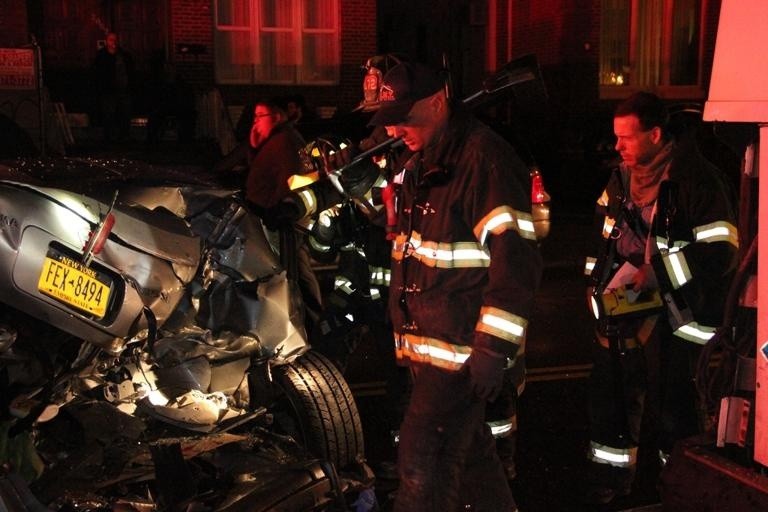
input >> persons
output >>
[66,30,740,512]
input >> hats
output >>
[365,56,450,129]
[350,53,405,114]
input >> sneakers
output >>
[572,480,634,503]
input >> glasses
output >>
[468,351,512,403]
